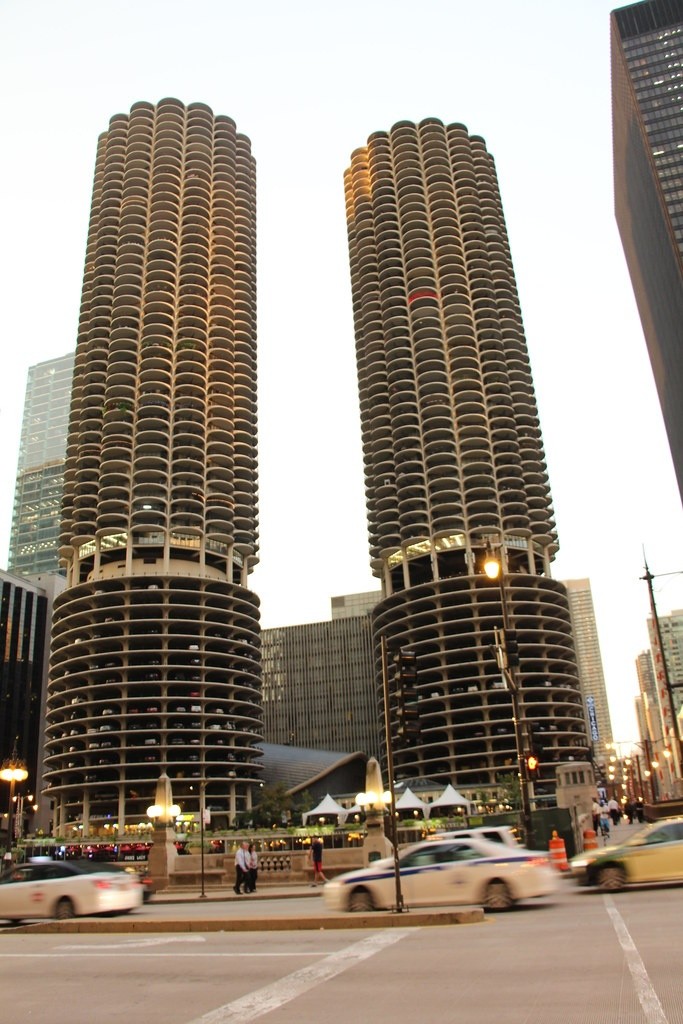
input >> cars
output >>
[568,815,683,897]
[321,836,562,913]
[0,859,144,922]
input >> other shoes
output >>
[323,879,329,884]
[312,883,316,887]
[243,885,248,892]
[233,886,241,894]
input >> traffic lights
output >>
[500,628,519,669]
[393,646,423,744]
[527,755,539,782]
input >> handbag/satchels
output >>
[616,814,619,821]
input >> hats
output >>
[600,800,605,805]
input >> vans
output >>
[422,826,528,851]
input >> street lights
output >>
[1,767,28,857]
[482,538,535,851]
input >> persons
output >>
[590,793,644,837]
[232,839,259,895]
[307,831,329,888]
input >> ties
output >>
[244,852,250,870]
